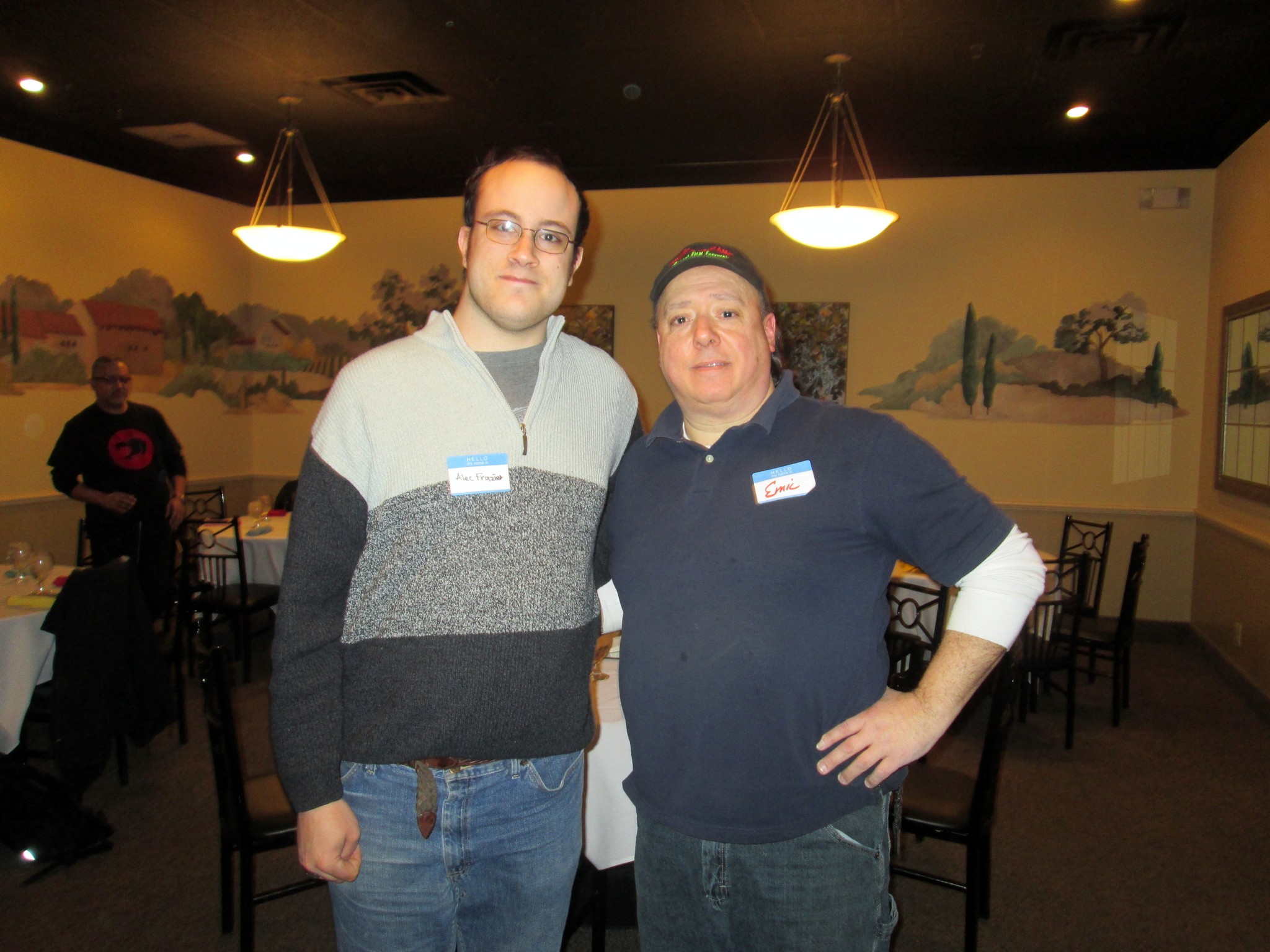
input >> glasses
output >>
[93,375,131,383]
[470,218,576,254]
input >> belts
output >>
[409,755,496,839]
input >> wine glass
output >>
[248,500,265,529]
[8,540,30,582]
[31,554,55,599]
[260,495,273,523]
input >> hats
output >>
[650,241,766,305]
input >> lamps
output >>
[764,52,900,252]
[228,95,348,264]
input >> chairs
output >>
[984,550,1093,753]
[1036,531,1152,729]
[1045,512,1116,686]
[885,572,949,769]
[887,646,1030,952]
[36,479,639,952]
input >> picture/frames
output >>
[1212,288,1270,507]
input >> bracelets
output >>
[172,494,185,504]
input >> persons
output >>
[45,355,186,569]
[591,240,1046,952]
[271,145,640,952]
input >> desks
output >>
[0,562,93,822]
[886,548,1063,675]
[197,510,294,650]
[577,579,638,923]
